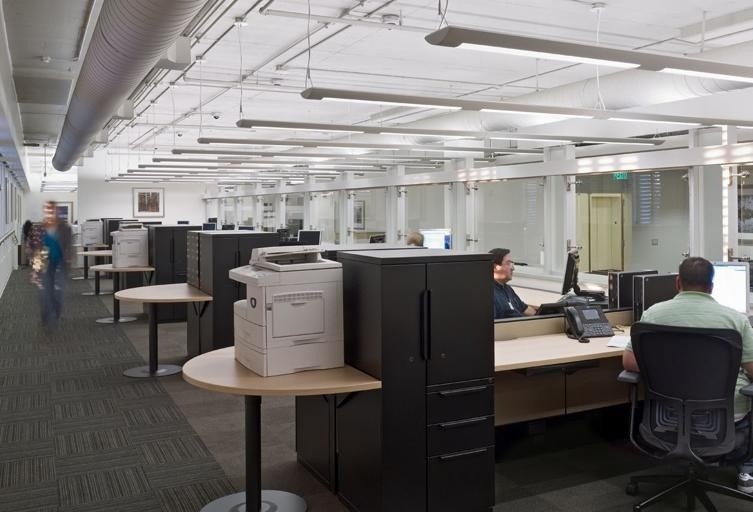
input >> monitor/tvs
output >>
[711,261,750,317]
[297,229,320,243]
[562,249,579,295]
[419,229,452,249]
[239,225,253,231]
[369,233,384,243]
[222,224,235,230]
[203,223,215,230]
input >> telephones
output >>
[563,305,615,338]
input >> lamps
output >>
[39,144,79,193]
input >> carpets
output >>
[0,267,240,512]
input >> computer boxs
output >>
[632,272,680,323]
[609,270,656,309]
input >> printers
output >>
[229,264,345,376]
[110,231,148,267]
[82,222,103,245]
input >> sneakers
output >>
[738,472,753,493]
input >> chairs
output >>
[616,320,753,512]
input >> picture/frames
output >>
[132,186,165,218]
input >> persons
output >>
[407,232,424,247]
[622,258,752,495]
[489,249,537,319]
[26,202,77,341]
